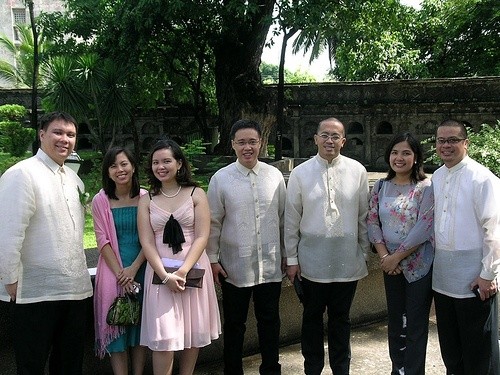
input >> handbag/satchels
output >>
[152,267,206,288]
[107,283,142,325]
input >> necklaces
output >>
[160,186,181,198]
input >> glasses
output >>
[318,132,344,142]
[233,140,261,145]
[435,136,464,144]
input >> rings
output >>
[126,277,129,281]
[393,271,396,274]
[493,293,496,295]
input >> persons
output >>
[431,120,500,375]
[91,146,148,375]
[367,132,435,375]
[207,120,287,375]
[137,139,222,375]
[284,118,370,375]
[0,112,93,375]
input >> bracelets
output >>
[162,273,172,284]
[381,253,390,259]
